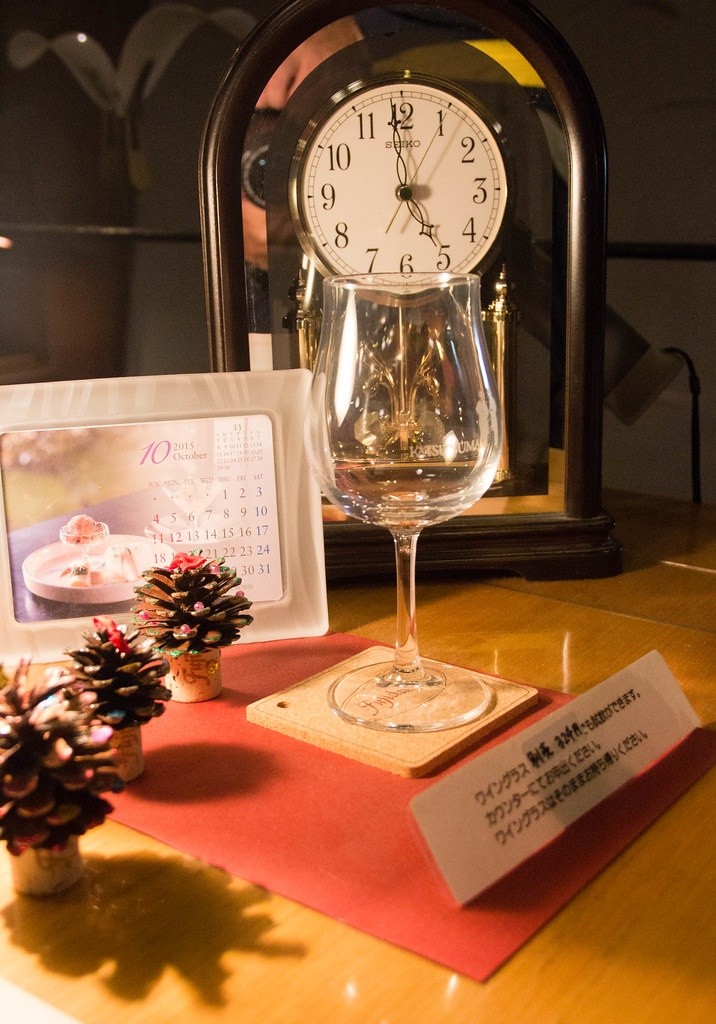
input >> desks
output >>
[0,481,716,1021]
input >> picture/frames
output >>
[0,368,329,667]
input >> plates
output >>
[22,534,177,605]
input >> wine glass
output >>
[307,273,500,734]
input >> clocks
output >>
[198,0,623,588]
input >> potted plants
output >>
[130,551,254,704]
[0,654,126,898]
[54,618,173,784]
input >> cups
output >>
[60,523,110,569]
